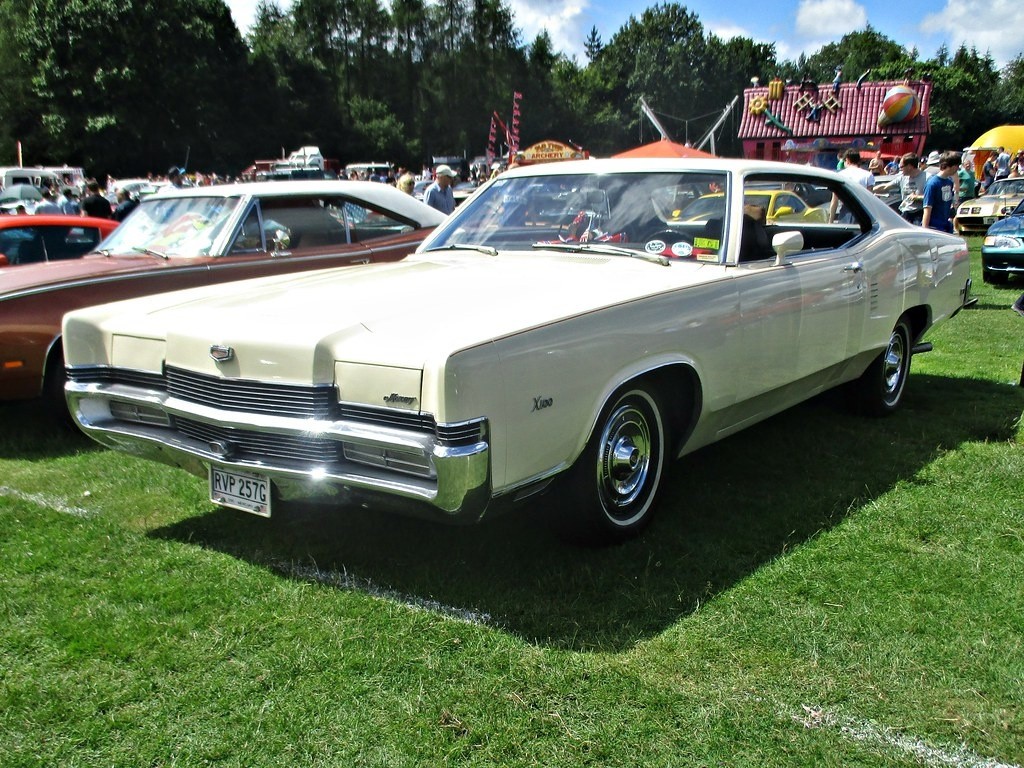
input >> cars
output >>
[743,180,818,208]
[0,214,121,268]
[809,175,903,224]
[0,179,573,434]
[667,190,831,227]
[982,199,1024,283]
[61,156,978,544]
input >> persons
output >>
[1,166,254,243]
[919,150,962,234]
[423,164,458,216]
[827,148,875,224]
[952,160,975,233]
[869,151,883,176]
[984,147,1024,195]
[336,165,415,196]
[884,155,900,175]
[836,151,845,170]
[873,152,934,226]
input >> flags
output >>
[514,92,523,100]
[513,102,520,151]
[488,116,496,156]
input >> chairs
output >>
[614,199,670,244]
[706,214,766,261]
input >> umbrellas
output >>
[0,184,42,201]
[611,138,720,160]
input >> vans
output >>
[344,163,394,177]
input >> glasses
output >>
[404,181,415,186]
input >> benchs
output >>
[670,226,861,253]
[297,230,398,248]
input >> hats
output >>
[925,151,941,165]
[168,167,186,180]
[435,164,458,178]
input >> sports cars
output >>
[953,175,1024,234]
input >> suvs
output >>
[0,166,81,200]
